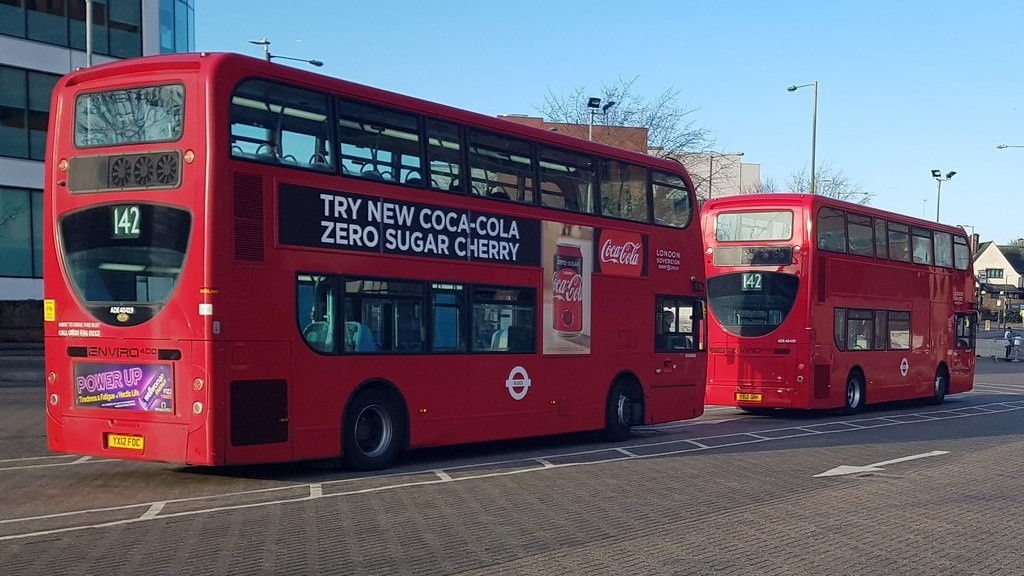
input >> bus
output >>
[698,193,980,415]
[43,54,710,472]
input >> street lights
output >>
[1003,274,1013,334]
[962,224,974,256]
[932,169,958,224]
[586,97,617,215]
[249,38,322,154]
[709,152,743,199]
[787,82,818,196]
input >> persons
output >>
[1004,327,1013,358]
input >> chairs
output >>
[231,143,512,202]
